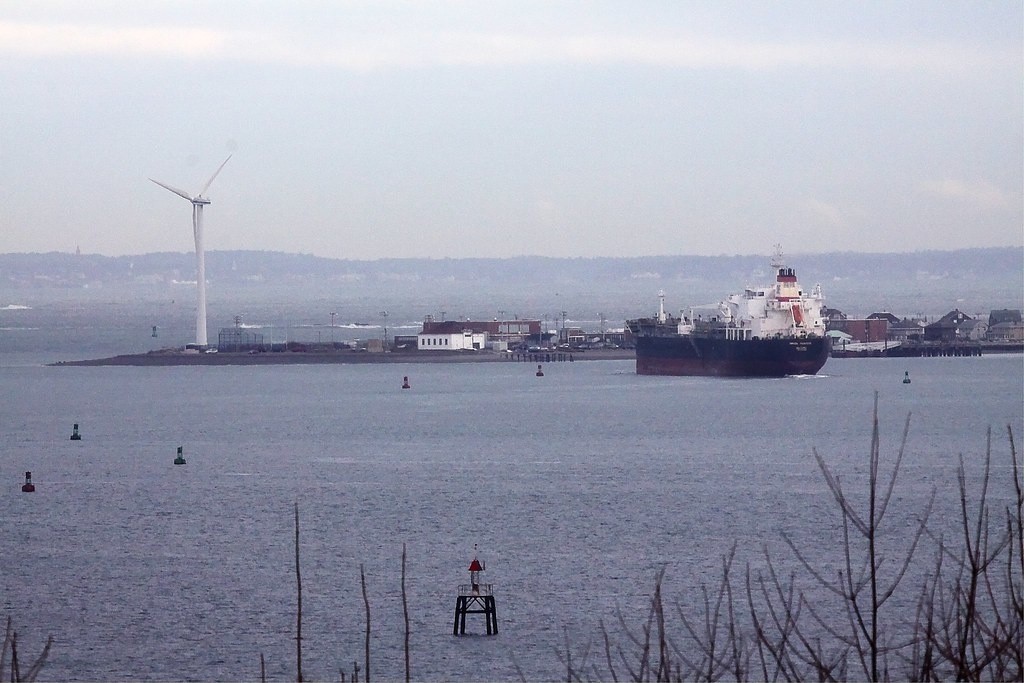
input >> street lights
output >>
[498,311,506,341]
[330,312,338,342]
[233,313,245,353]
[382,310,390,345]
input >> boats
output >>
[637,246,833,379]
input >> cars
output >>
[511,340,620,353]
